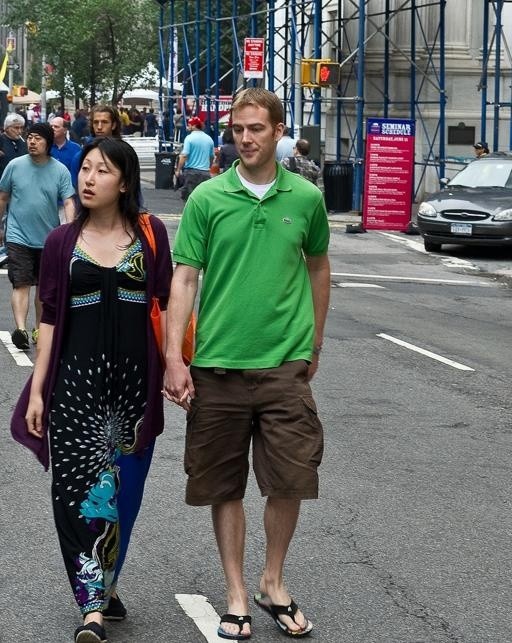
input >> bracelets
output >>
[311,344,322,357]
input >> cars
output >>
[417,152,510,260]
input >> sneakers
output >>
[0,248,9,267]
[13,327,39,349]
[74,595,126,641]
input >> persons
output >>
[280,138,326,194]
[48,116,82,225]
[165,86,332,639]
[0,122,77,349]
[473,142,489,157]
[275,126,295,167]
[215,128,241,173]
[9,136,192,642]
[173,116,216,204]
[0,112,28,269]
[51,102,184,144]
[70,102,145,215]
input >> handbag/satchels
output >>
[210,164,220,177]
[174,174,184,190]
[151,295,196,364]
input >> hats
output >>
[189,118,203,129]
[473,142,488,149]
[27,122,54,145]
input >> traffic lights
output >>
[315,60,343,87]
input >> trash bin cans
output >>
[155,152,176,189]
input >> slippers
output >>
[254,591,314,638]
[217,614,251,639]
